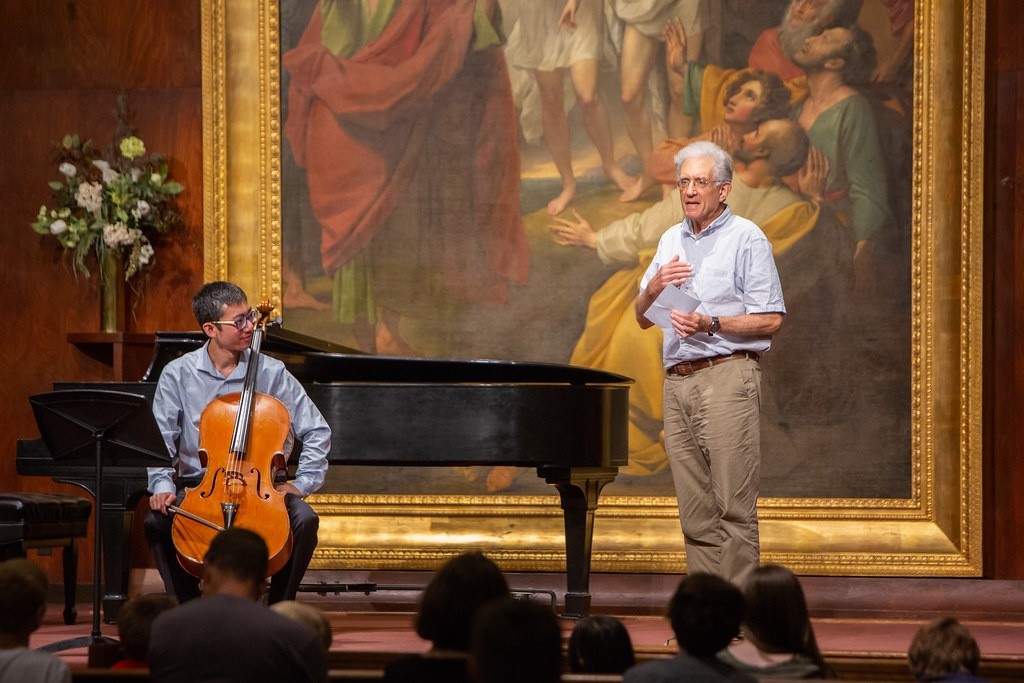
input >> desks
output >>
[68,332,156,383]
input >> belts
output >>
[668,350,756,375]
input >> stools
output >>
[1,492,92,624]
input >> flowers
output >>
[29,131,186,324]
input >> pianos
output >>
[27,318,636,619]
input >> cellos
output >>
[170,300,294,603]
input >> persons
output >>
[622,573,761,683]
[416,552,563,683]
[146,281,331,608]
[568,616,637,676]
[111,593,180,671]
[150,528,334,683]
[908,617,986,683]
[716,565,828,680]
[0,557,71,683]
[636,141,786,645]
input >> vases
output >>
[101,248,119,331]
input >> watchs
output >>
[708,317,720,337]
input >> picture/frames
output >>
[202,0,986,578]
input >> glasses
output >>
[212,309,258,329]
[677,178,725,191]
